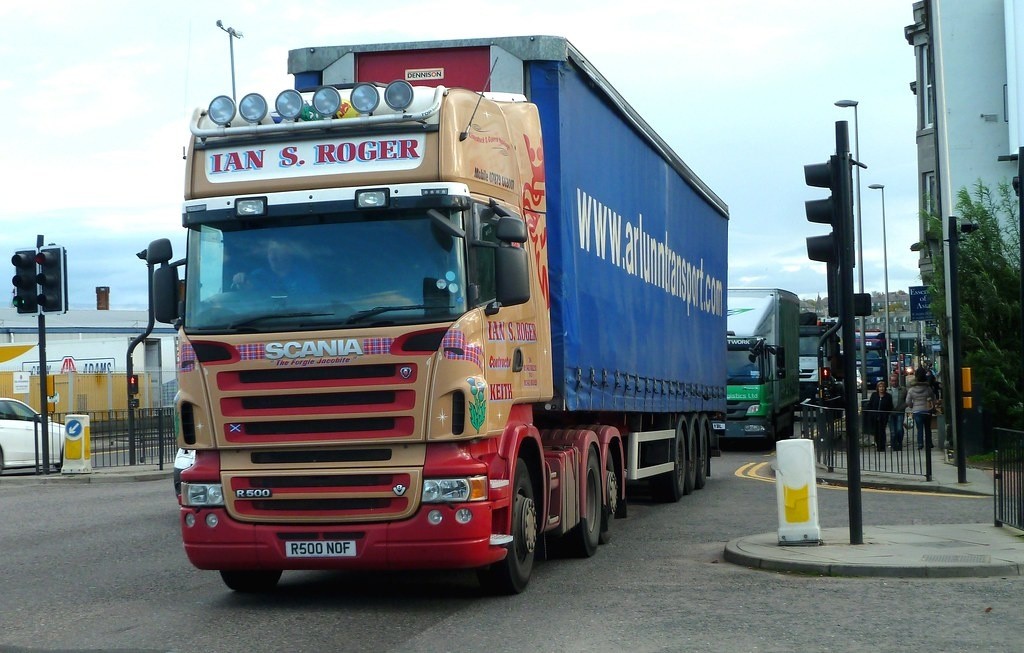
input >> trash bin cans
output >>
[61,415,92,474]
[775,438,825,547]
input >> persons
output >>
[905,373,936,450]
[885,376,907,451]
[232,232,309,297]
[904,366,939,400]
[868,381,892,451]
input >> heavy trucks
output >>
[145,34,729,596]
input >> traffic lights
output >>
[822,367,830,379]
[36,245,65,317]
[128,374,140,396]
[11,249,39,314]
[803,152,843,265]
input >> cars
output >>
[0,396,65,476]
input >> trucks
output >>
[720,287,804,451]
[794,308,920,421]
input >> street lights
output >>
[868,181,895,391]
[833,99,868,401]
[945,215,981,486]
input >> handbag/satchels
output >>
[926,392,936,414]
[903,413,914,429]
